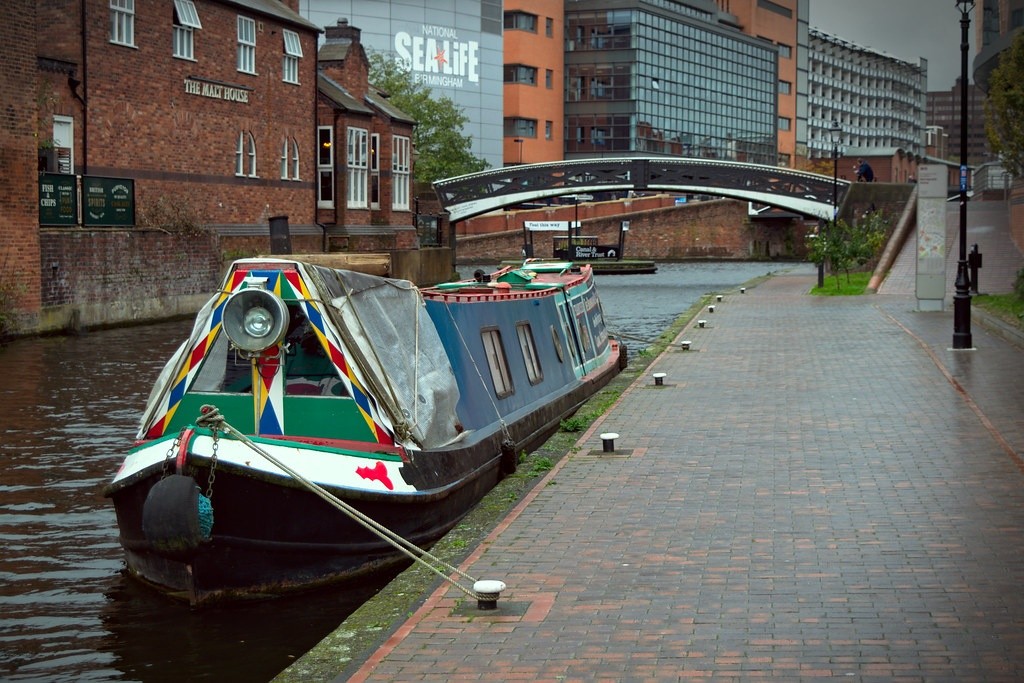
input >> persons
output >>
[853,158,874,182]
[908,176,917,183]
[865,203,877,237]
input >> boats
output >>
[103,254,626,625]
[496,196,658,277]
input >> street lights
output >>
[514,139,523,165]
[828,118,842,229]
[955,1,973,350]
